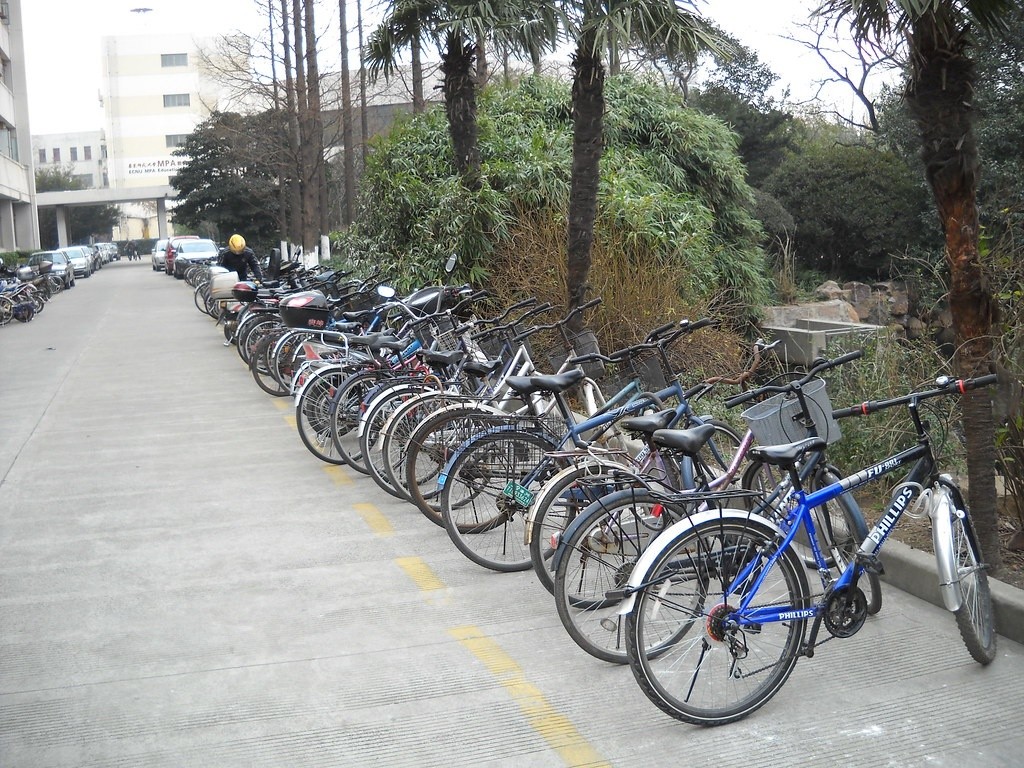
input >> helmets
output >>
[229,234,246,254]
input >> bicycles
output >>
[614,372,1003,728]
[127,244,138,261]
[184,246,785,611]
[548,349,885,666]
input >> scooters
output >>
[0,258,64,327]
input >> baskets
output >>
[435,314,480,351]
[544,331,606,379]
[349,294,388,311]
[414,314,458,348]
[477,323,533,360]
[600,354,666,406]
[741,379,842,445]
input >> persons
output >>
[124,239,141,259]
[216,234,262,284]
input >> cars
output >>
[27,250,76,290]
[170,238,225,280]
[151,239,169,272]
[57,242,121,278]
[160,235,201,275]
